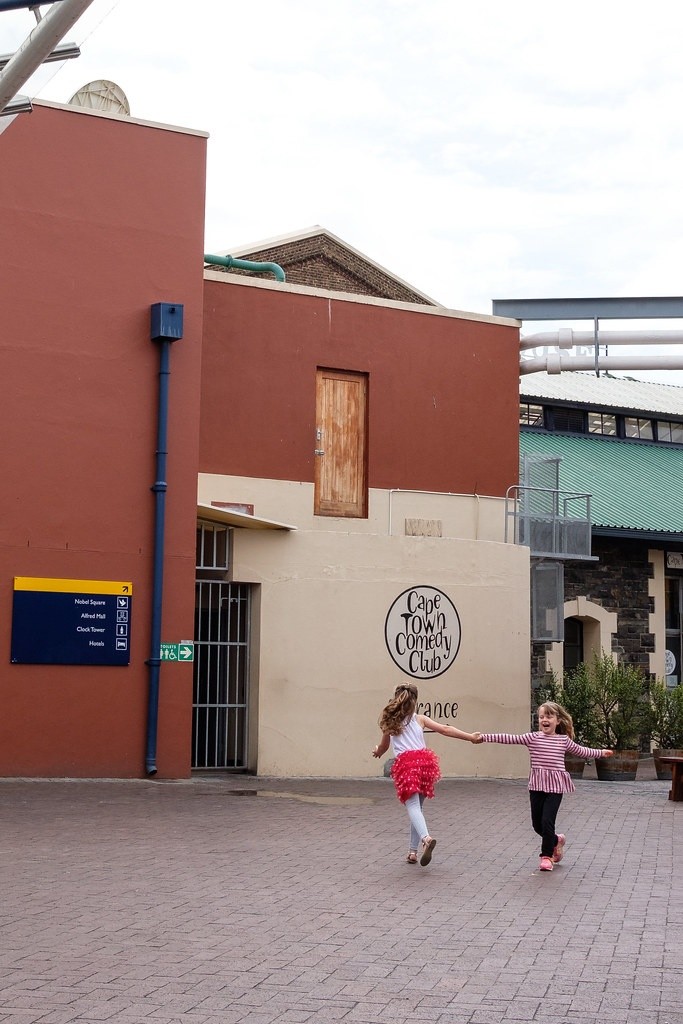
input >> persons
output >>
[372,684,482,866]
[477,701,613,870]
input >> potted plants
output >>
[531,661,588,779]
[650,674,683,781]
[588,651,650,782]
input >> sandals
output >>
[407,850,418,863]
[419,835,437,866]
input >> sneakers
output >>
[553,834,566,862]
[539,856,553,871]
